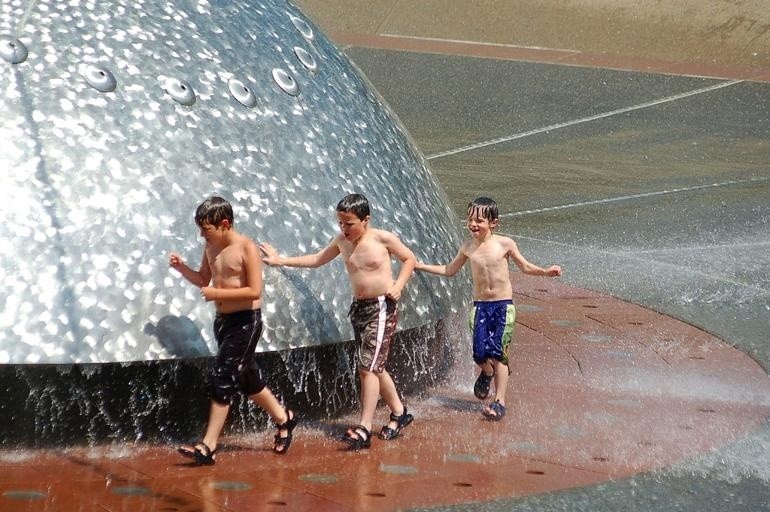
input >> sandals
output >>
[379,406,413,440]
[474,362,495,399]
[483,402,504,421]
[274,408,297,453]
[177,442,217,465]
[342,425,372,449]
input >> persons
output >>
[167,196,299,466]
[257,193,417,449]
[414,196,563,421]
